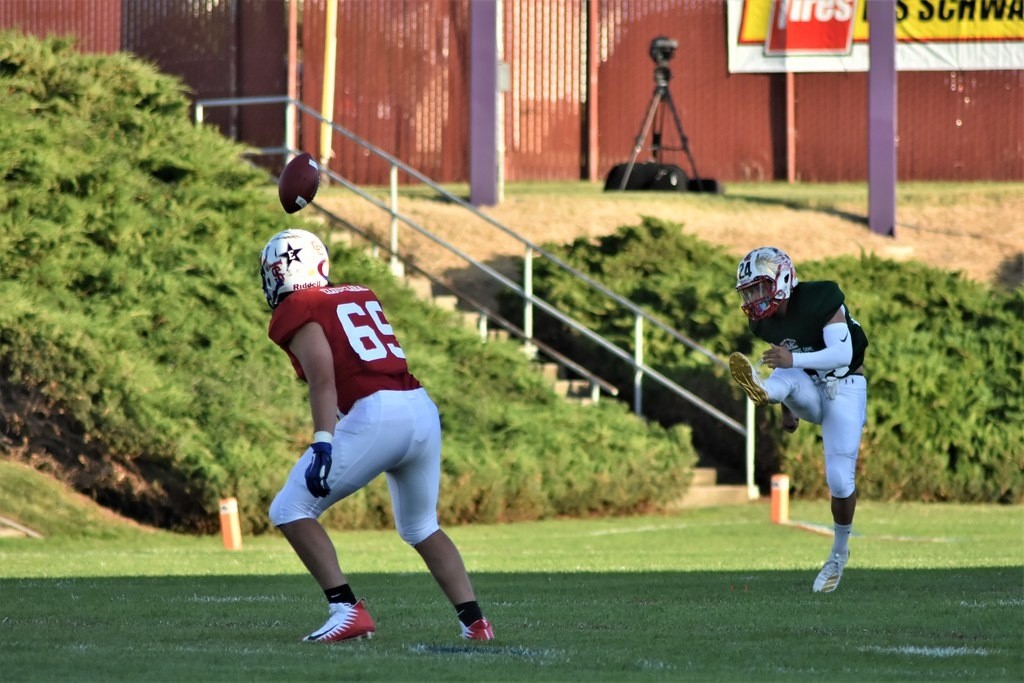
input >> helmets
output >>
[736,247,798,299]
[262,229,329,309]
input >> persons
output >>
[729,246,868,594]
[260,230,494,645]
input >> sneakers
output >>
[730,352,769,407]
[458,617,494,640]
[301,600,375,642]
[813,549,850,594]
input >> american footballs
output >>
[277,152,320,213]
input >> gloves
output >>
[305,441,333,498]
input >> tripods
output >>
[619,68,706,193]
